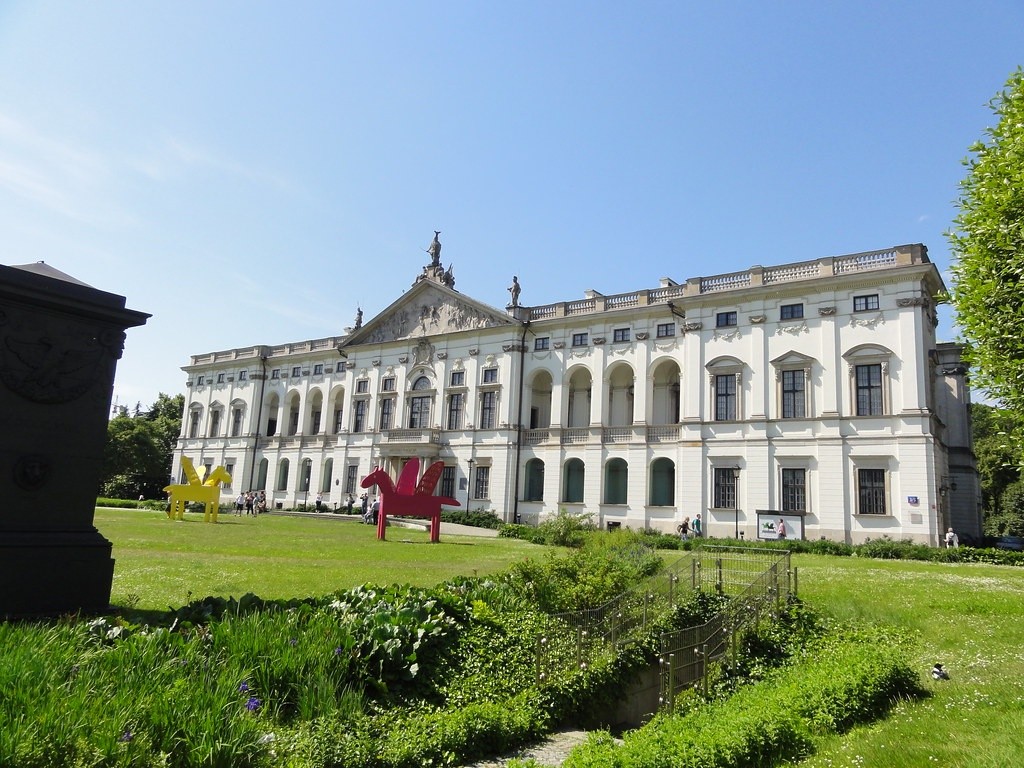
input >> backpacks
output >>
[692,520,700,530]
[948,535,954,546]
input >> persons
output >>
[693,514,700,538]
[360,493,368,514]
[416,237,455,289]
[680,517,693,540]
[778,519,786,540]
[316,492,323,513]
[354,308,363,329]
[511,276,521,305]
[370,499,380,525]
[139,492,145,501]
[235,491,266,516]
[346,494,355,515]
[166,492,172,518]
[944,528,959,549]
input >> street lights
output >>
[732,464,742,539]
[304,476,310,512]
[466,458,474,517]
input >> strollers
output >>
[363,508,374,524]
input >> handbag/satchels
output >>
[352,500,355,503]
[245,502,249,507]
[677,524,682,533]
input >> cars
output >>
[996,536,1024,551]
